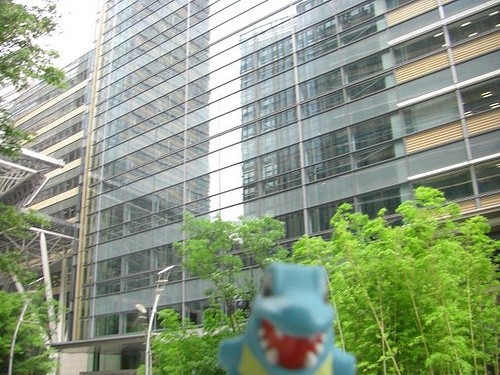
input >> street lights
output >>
[8,276,43,374]
[145,265,176,375]
